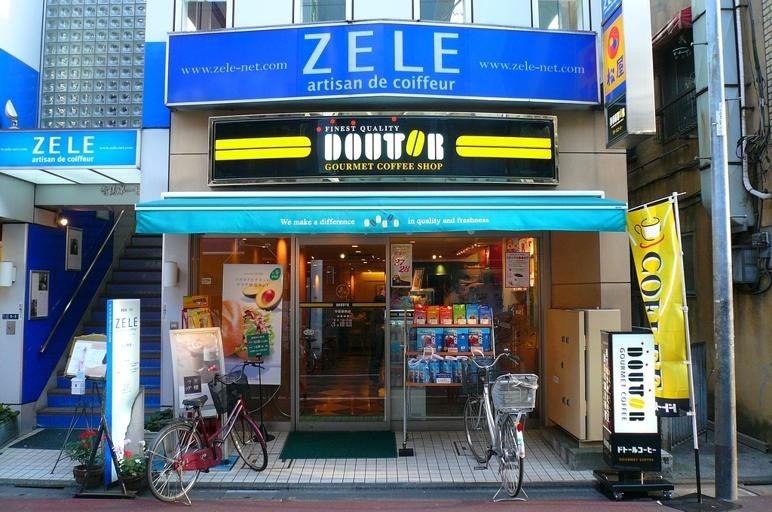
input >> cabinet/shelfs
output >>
[399,306,495,458]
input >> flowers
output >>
[66,431,105,468]
[121,451,149,477]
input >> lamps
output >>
[435,265,447,275]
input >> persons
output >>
[366,295,386,383]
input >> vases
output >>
[123,476,145,493]
[74,465,105,488]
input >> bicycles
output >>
[146,361,268,506]
[302,334,320,373]
[461,348,529,503]
[272,344,307,418]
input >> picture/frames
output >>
[29,270,50,320]
[65,226,83,271]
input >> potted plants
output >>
[0,401,19,448]
[143,409,173,472]
